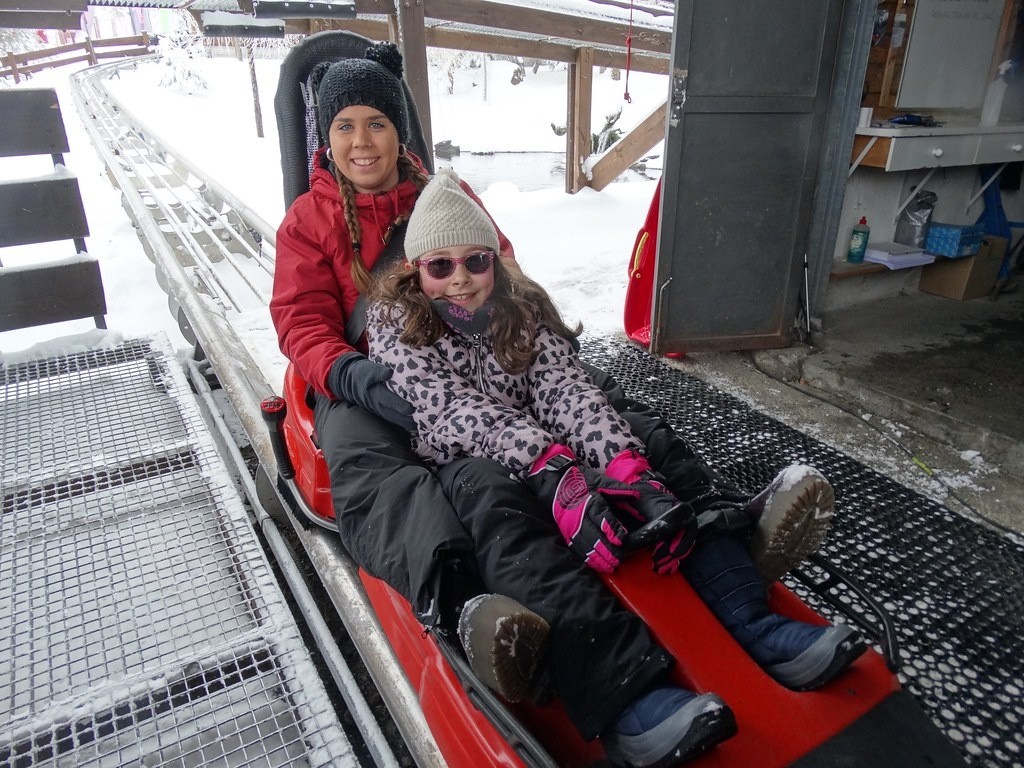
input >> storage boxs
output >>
[919,222,1010,302]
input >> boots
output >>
[608,682,738,768]
[690,554,867,689]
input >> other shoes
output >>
[457,593,550,710]
[743,463,832,590]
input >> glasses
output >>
[412,250,495,280]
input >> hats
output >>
[404,164,500,261]
[313,41,408,146]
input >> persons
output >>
[269,41,835,707]
[365,169,868,768]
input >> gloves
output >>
[329,352,415,429]
[523,444,640,576]
[599,447,699,577]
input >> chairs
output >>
[261,30,439,533]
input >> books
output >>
[866,239,936,273]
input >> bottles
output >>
[846,216,870,263]
